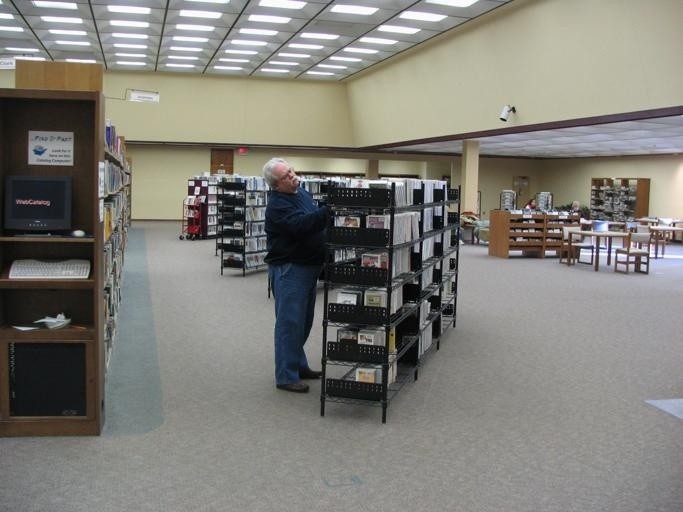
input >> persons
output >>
[258,157,338,393]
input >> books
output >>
[184,173,239,243]
[296,173,322,206]
[220,175,271,269]
[500,185,675,243]
[320,173,453,386]
[99,119,131,374]
[12,325,41,332]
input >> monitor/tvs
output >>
[3,176,72,238]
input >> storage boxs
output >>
[13,58,104,93]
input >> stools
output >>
[461,221,475,244]
[476,226,490,246]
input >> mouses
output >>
[71,229,86,238]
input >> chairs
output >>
[558,217,682,274]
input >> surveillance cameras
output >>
[499,105,515,122]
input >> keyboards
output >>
[8,258,90,279]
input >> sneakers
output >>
[300,368,322,379]
[276,382,309,392]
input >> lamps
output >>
[498,103,517,123]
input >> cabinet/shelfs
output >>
[0,87,135,437]
[311,178,462,423]
[176,171,217,245]
[588,175,650,221]
[485,206,581,263]
[212,174,312,277]
[295,170,422,181]
